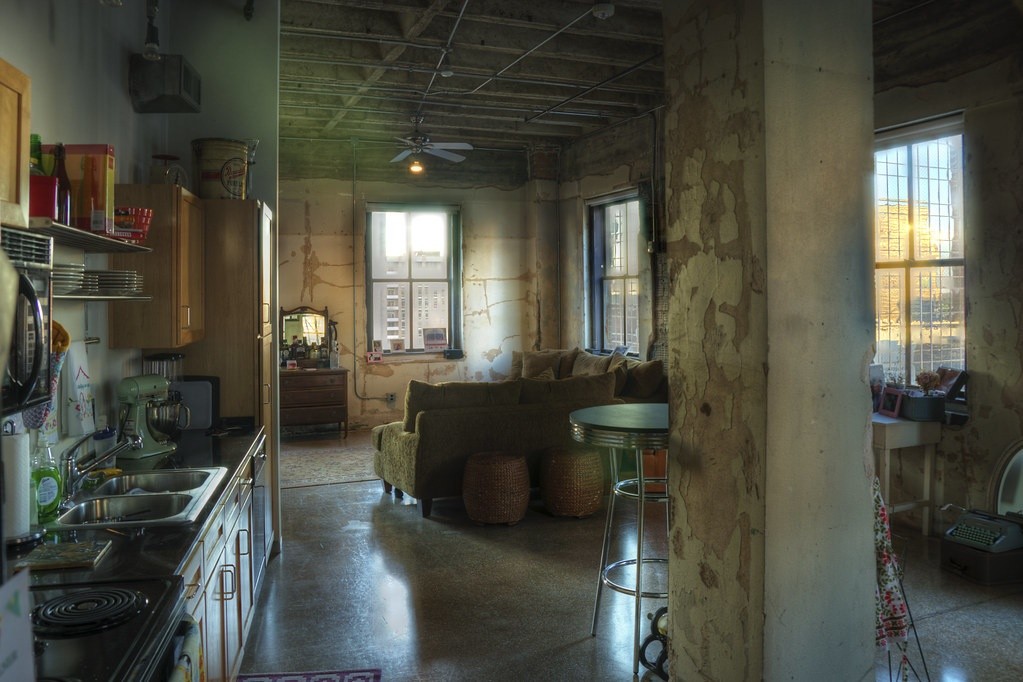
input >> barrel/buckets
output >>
[192,138,249,201]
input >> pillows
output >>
[400,346,664,432]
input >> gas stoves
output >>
[0,546,187,681]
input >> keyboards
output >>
[949,522,1003,546]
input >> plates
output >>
[52,264,144,295]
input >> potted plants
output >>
[885,371,907,389]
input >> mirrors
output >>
[282,313,326,347]
[986,437,1023,516]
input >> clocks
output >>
[164,162,194,192]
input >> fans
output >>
[351,114,474,163]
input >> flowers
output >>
[916,371,940,391]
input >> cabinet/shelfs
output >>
[109,182,276,567]
[279,306,351,439]
[178,432,276,682]
[0,57,32,231]
[28,217,155,301]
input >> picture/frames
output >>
[366,352,382,363]
[878,388,903,417]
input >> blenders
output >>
[113,374,180,458]
[139,352,191,436]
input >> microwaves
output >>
[1,257,52,414]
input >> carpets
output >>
[236,668,382,682]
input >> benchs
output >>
[370,375,668,518]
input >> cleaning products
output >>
[29,434,63,524]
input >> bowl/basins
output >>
[113,206,154,246]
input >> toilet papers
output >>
[0,433,30,538]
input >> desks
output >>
[871,411,941,537]
[569,403,669,675]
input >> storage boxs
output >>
[40,144,115,233]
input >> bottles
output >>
[281,334,340,370]
[77,154,100,233]
[48,142,72,228]
[30,134,47,178]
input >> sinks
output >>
[45,493,213,529]
[82,466,228,493]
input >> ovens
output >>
[134,610,206,682]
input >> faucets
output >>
[58,429,144,500]
